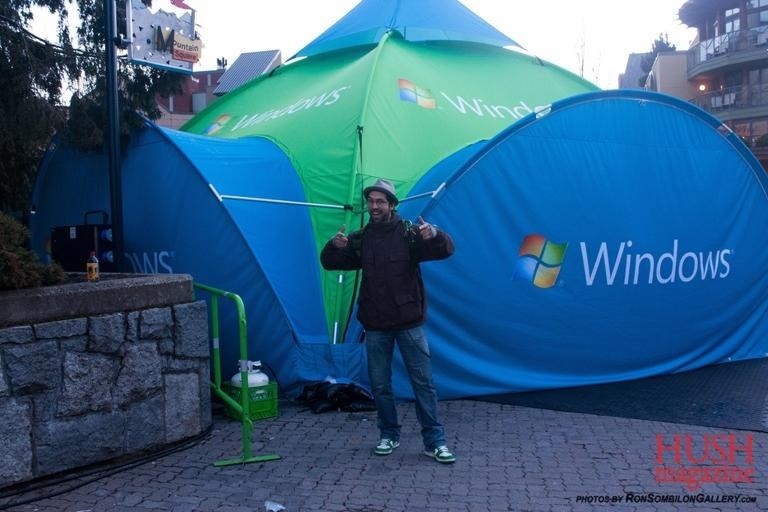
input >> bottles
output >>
[87,252,100,282]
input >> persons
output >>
[319,176,462,466]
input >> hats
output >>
[364,179,399,206]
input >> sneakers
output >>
[374,438,400,455]
[424,445,455,463]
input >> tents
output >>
[23,0,767,410]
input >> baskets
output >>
[224,380,279,421]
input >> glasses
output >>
[367,198,386,207]
